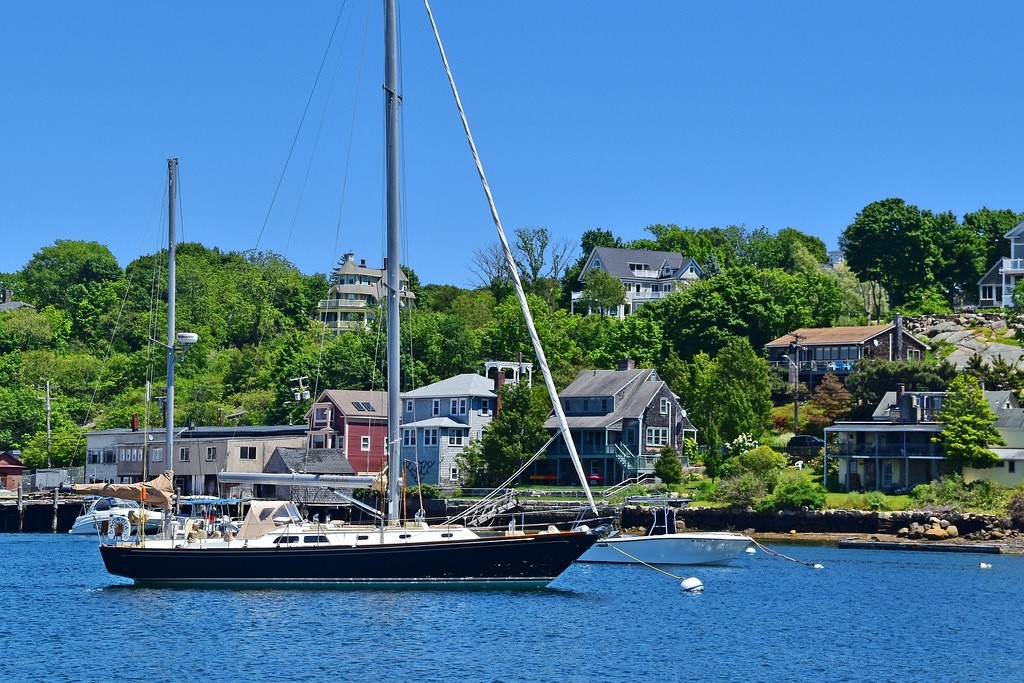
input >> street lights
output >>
[782,354,798,424]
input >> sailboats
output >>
[100,0,621,592]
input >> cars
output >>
[787,435,824,447]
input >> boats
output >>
[71,495,164,533]
